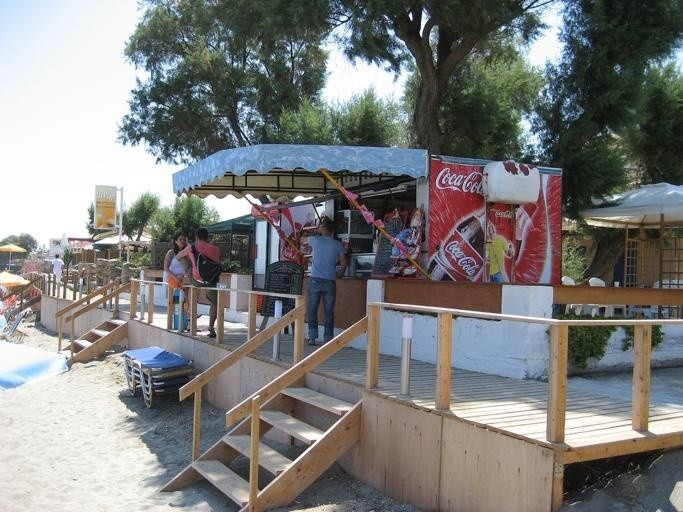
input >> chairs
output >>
[0,311,28,344]
[561,275,628,317]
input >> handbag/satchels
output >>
[194,252,222,284]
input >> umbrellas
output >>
[0,271,31,286]
[0,244,27,270]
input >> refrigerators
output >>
[252,202,315,313]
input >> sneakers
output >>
[187,328,201,332]
[209,329,216,338]
[182,280,191,284]
[309,338,315,344]
[188,314,202,321]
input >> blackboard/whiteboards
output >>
[261,261,305,317]
[371,219,402,278]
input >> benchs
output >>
[120,347,196,408]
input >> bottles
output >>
[428,202,538,282]
[281,221,302,264]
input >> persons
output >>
[301,216,348,345]
[163,230,202,332]
[52,254,65,282]
[176,227,221,337]
